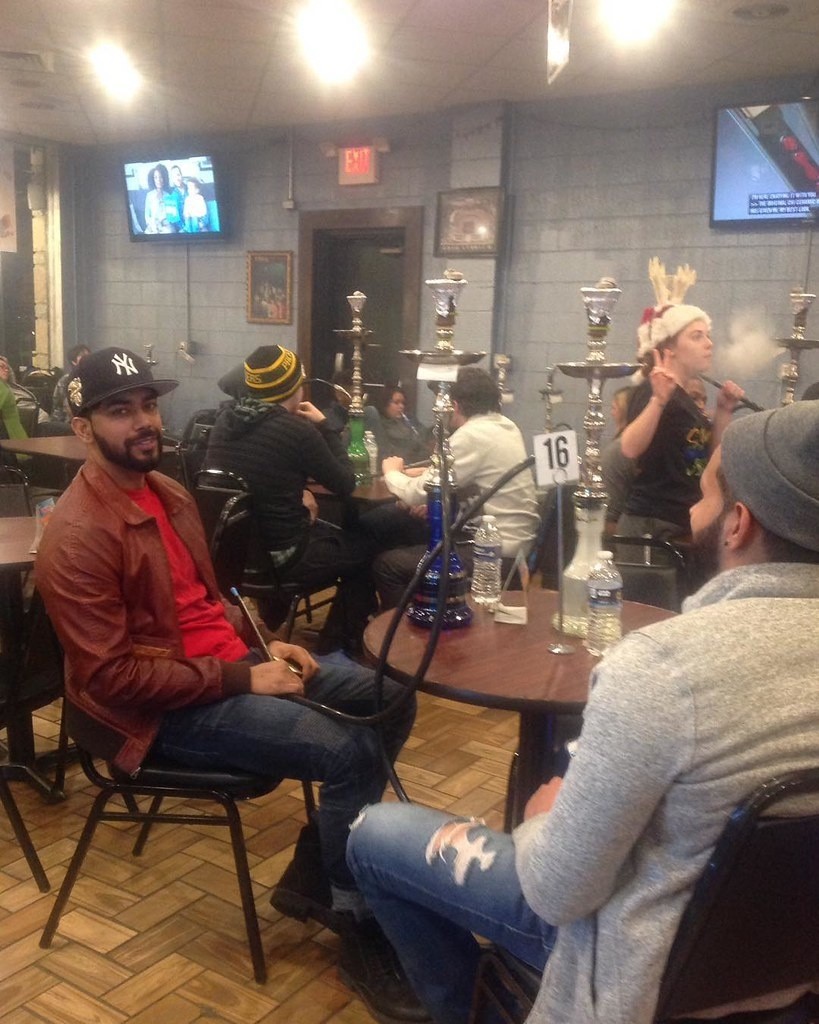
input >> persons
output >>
[345,401,819,1023]
[144,164,209,235]
[0,301,545,609]
[34,346,434,1024]
[595,256,819,616]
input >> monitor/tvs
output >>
[709,96,819,229]
[119,152,224,242]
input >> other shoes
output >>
[317,641,375,670]
[336,943,431,1024]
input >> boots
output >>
[271,828,348,936]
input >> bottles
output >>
[586,550,623,657]
[363,431,377,474]
[470,514,502,603]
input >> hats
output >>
[244,345,304,402]
[65,347,179,417]
[720,399,819,552]
[637,305,711,357]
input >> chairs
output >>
[0,367,818,1024]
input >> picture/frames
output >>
[246,250,292,325]
[434,187,504,258]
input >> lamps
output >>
[318,137,391,186]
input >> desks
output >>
[0,517,40,571]
[0,436,179,462]
[300,477,400,531]
[362,587,684,838]
